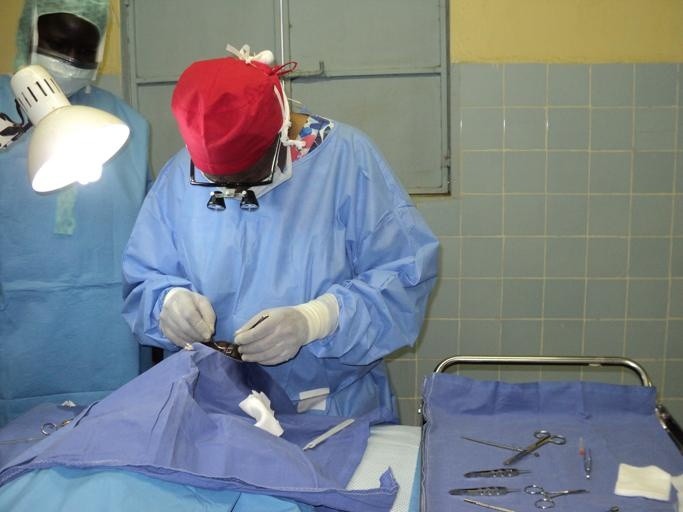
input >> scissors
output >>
[503,430,566,466]
[524,484,589,510]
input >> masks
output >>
[19,8,103,99]
[196,142,293,199]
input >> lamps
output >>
[0,65,130,193]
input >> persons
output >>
[0,0,154,397]
[118,42,441,426]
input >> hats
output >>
[171,57,288,176]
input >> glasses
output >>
[189,144,281,191]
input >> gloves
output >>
[158,287,217,347]
[233,294,340,367]
